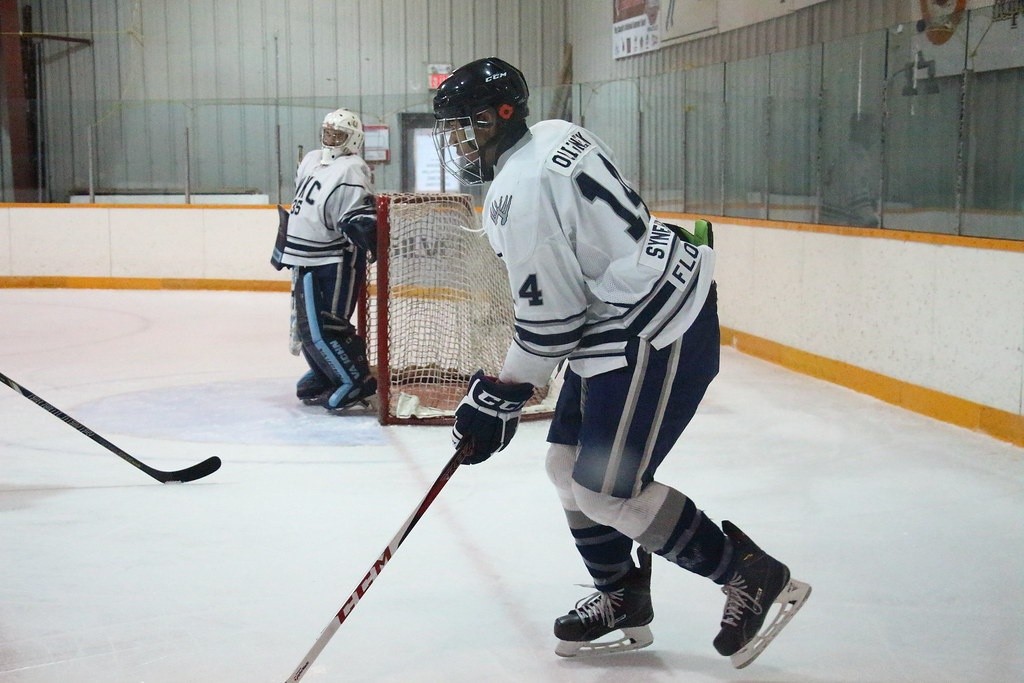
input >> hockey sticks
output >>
[0,371,221,485]
[271,435,471,683]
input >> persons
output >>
[286,106,379,411]
[816,113,882,228]
[430,58,812,670]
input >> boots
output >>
[294,330,377,414]
[554,545,655,659]
[714,520,811,669]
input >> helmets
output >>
[434,56,528,132]
[320,107,364,165]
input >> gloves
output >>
[451,371,536,465]
[349,217,379,263]
